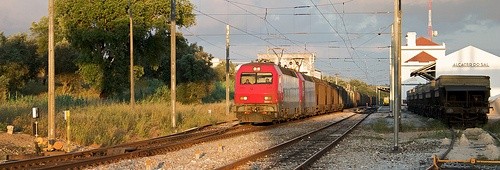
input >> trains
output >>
[233,62,384,125]
[406,75,491,124]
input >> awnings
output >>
[377,87,390,93]
[410,61,435,82]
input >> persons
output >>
[254,57,271,63]
[244,79,251,84]
[294,60,303,72]
[284,64,288,68]
[288,62,295,69]
[277,62,282,67]
